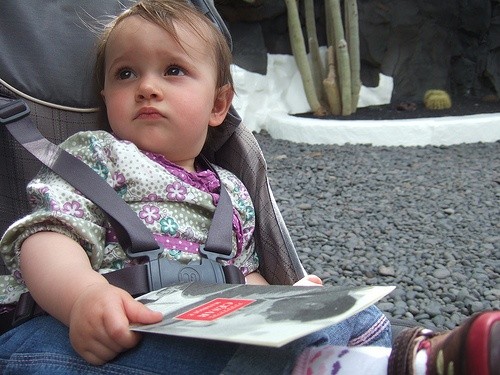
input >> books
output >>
[127,282,396,347]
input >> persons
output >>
[0,0,500,375]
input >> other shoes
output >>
[388,306,500,375]
